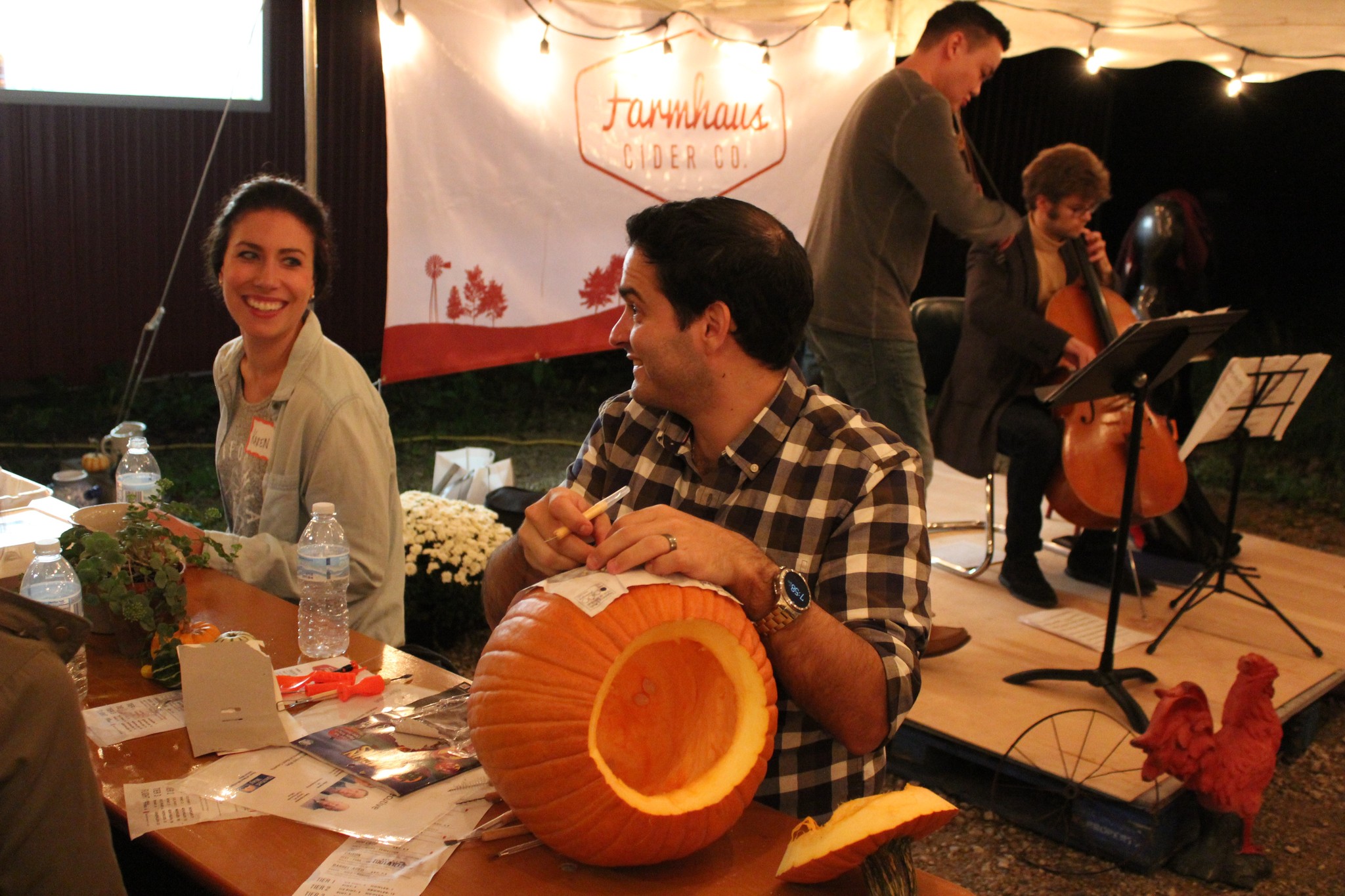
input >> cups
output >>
[49,469,97,509]
[100,420,146,466]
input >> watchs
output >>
[753,566,813,637]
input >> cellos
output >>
[1035,224,1192,533]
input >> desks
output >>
[0,548,979,896]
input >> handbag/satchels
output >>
[430,446,515,505]
[485,486,550,532]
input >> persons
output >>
[795,0,1024,656]
[129,174,408,655]
[1,626,129,896]
[932,141,1158,609]
[481,196,936,826]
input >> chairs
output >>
[909,294,1016,582]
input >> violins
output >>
[946,109,1003,219]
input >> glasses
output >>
[1057,200,1100,218]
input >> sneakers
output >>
[999,544,1061,608]
[917,624,970,660]
[1063,525,1159,597]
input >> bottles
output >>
[114,435,162,511]
[20,538,89,705]
[297,503,349,660]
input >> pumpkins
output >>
[82,450,110,471]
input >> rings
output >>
[661,534,677,553]
